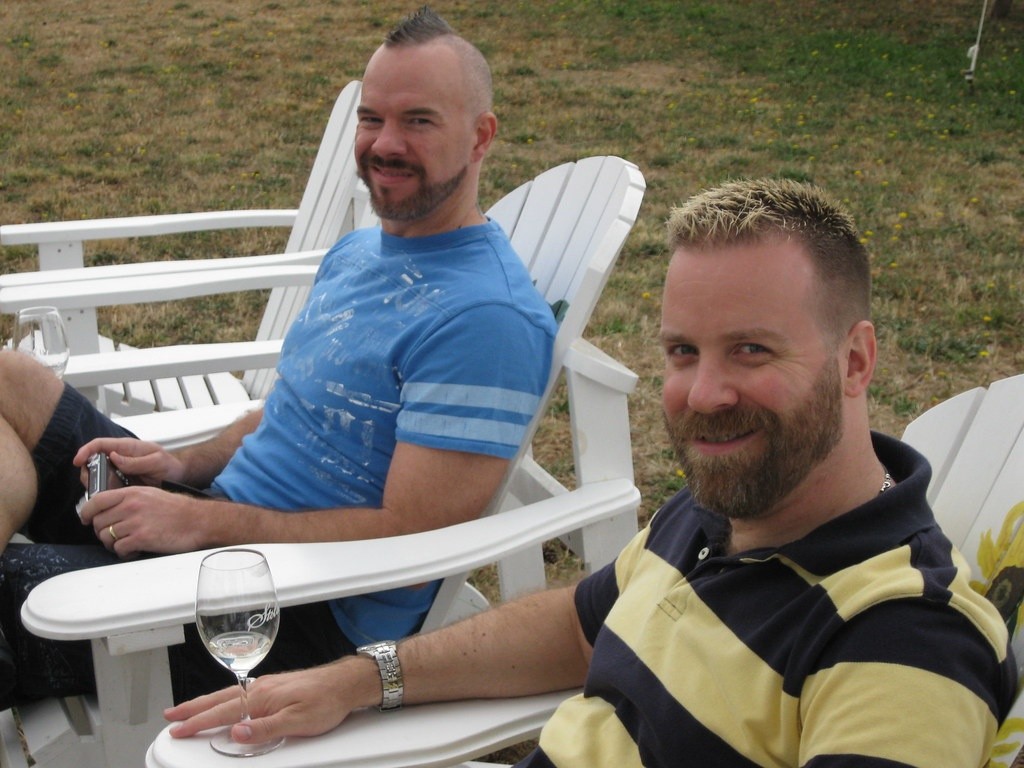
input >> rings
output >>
[110,526,118,540]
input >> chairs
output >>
[145,371,1024,768]
[0,79,384,420]
[0,154,644,768]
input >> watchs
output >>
[357,639,403,708]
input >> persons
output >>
[0,7,557,717]
[167,175,1021,767]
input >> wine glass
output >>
[194,549,286,757]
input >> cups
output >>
[12,305,71,380]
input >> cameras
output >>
[86,452,131,501]
